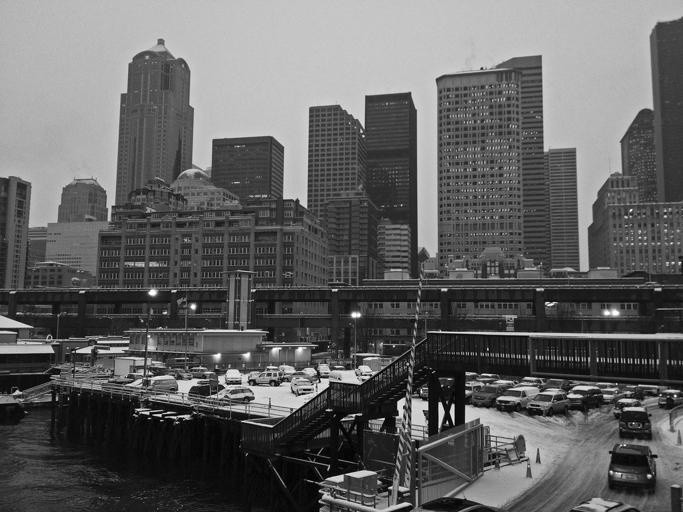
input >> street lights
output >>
[183,302,197,369]
[351,311,361,368]
[55,311,66,339]
[142,288,158,377]
[421,370,667,417]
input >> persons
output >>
[317,370,321,382]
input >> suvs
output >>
[618,405,653,439]
[607,442,658,494]
[290,376,314,396]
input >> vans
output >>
[328,370,362,390]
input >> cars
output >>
[301,367,317,380]
[657,389,682,408]
[79,361,294,409]
[568,495,639,512]
[409,496,502,512]
[287,371,312,383]
[317,364,330,377]
[354,364,371,376]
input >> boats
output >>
[0,390,24,417]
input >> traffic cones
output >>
[535,447,541,464]
[493,453,500,469]
[524,457,533,477]
